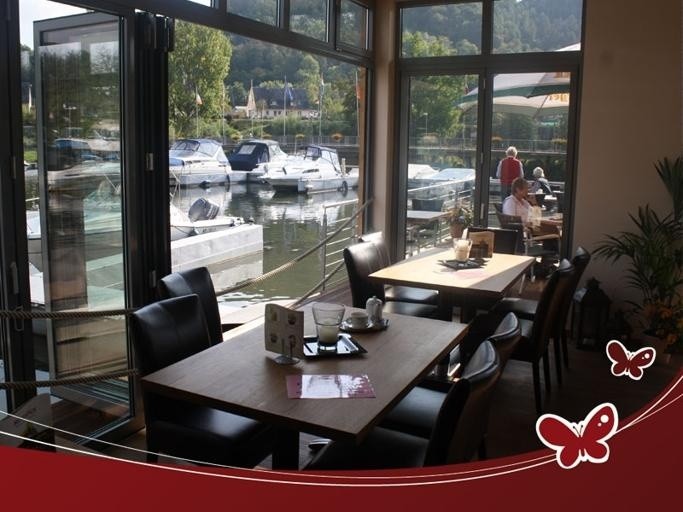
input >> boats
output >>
[169,184,344,225]
[45,159,120,193]
[46,137,120,170]
[86,121,120,142]
[228,138,287,171]
[413,167,476,191]
[24,158,248,271]
[407,164,439,189]
[168,137,247,185]
[407,180,465,200]
[255,144,344,192]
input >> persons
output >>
[497,146,524,201]
[528,167,551,194]
[502,178,557,279]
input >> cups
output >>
[451,237,474,262]
[311,300,345,350]
[268,332,278,343]
[287,313,296,324]
[288,334,296,347]
[270,311,277,321]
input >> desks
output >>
[141,301,470,472]
[368,240,537,346]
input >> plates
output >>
[341,320,374,329]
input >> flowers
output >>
[591,155,683,355]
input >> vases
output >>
[643,330,672,365]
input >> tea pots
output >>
[344,311,369,330]
[365,294,383,324]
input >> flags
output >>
[284,78,293,101]
[320,76,324,97]
[197,93,202,104]
[465,82,469,94]
[356,75,360,98]
[246,82,256,117]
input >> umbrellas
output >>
[458,40,582,113]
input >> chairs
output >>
[490,267,573,409]
[303,379,470,470]
[343,241,441,322]
[377,311,521,460]
[496,246,589,384]
[129,294,272,469]
[358,229,439,303]
[161,268,222,345]
